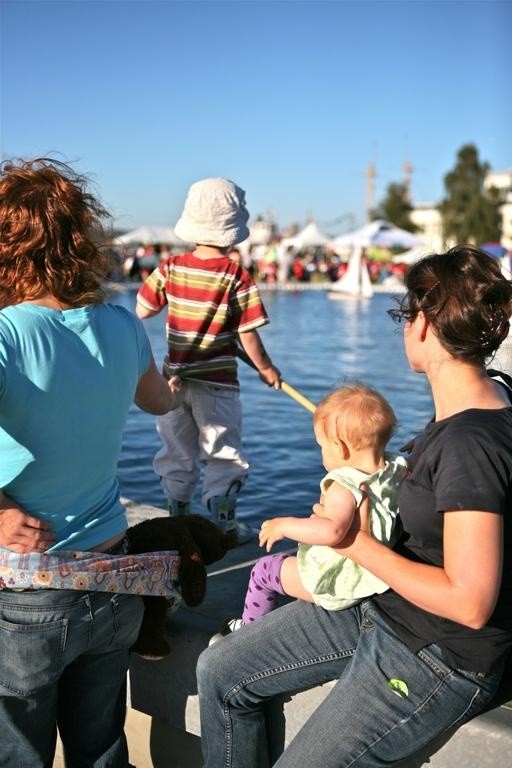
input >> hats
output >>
[174,176,250,248]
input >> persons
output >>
[229,245,408,284]
[133,171,286,551]
[206,378,415,646]
[120,242,172,284]
[193,241,512,767]
[1,154,181,766]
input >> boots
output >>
[208,493,253,545]
